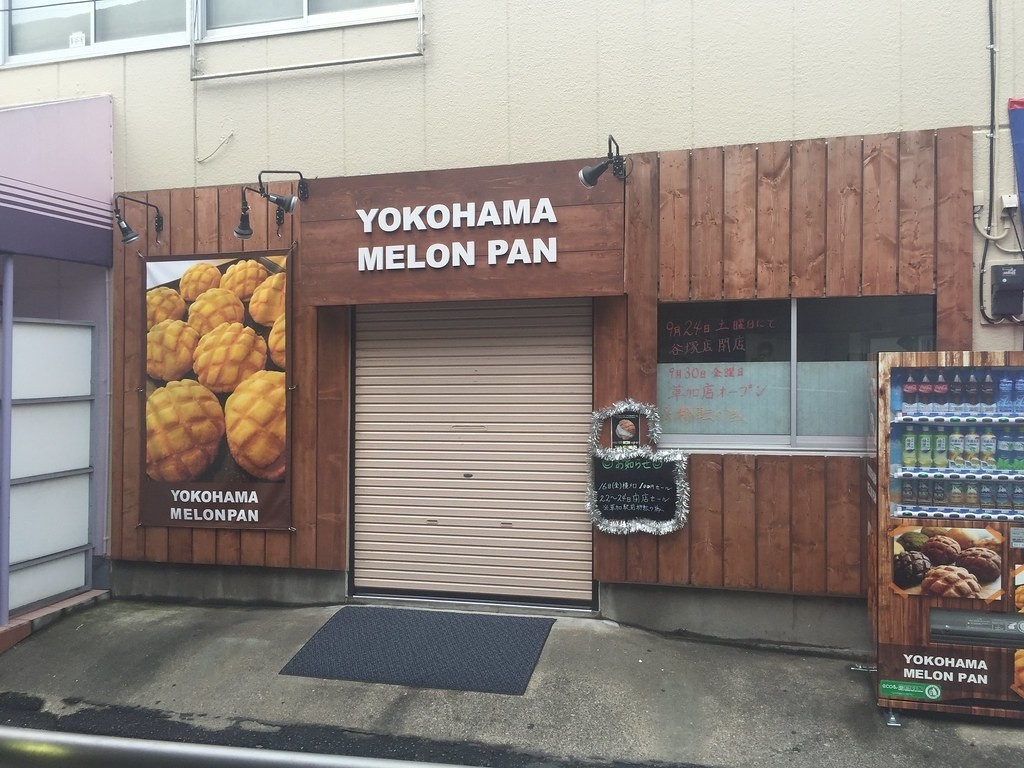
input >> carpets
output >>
[277,606,556,696]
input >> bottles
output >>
[898,368,1024,471]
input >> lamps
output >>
[258,171,307,215]
[577,135,622,189]
[113,195,162,244]
[232,187,283,240]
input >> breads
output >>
[1014,585,1024,611]
[146,259,285,484]
[891,527,1003,599]
[1013,649,1024,699]
